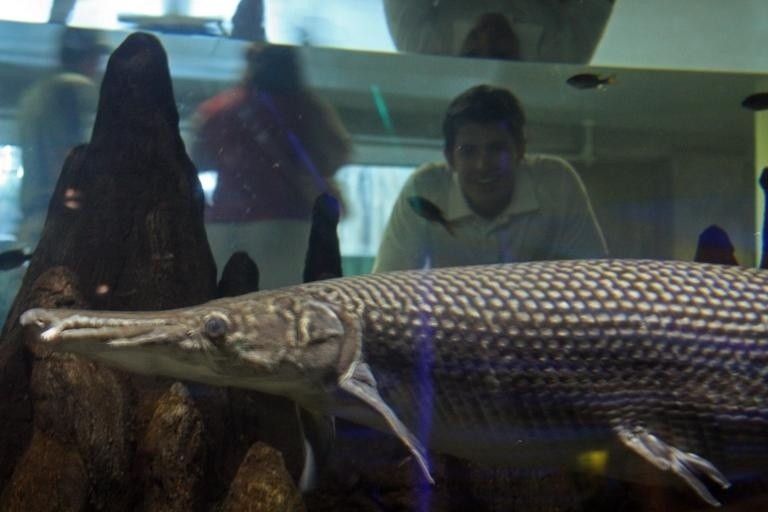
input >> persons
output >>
[178,46,354,285]
[372,81,613,280]
[14,28,110,254]
